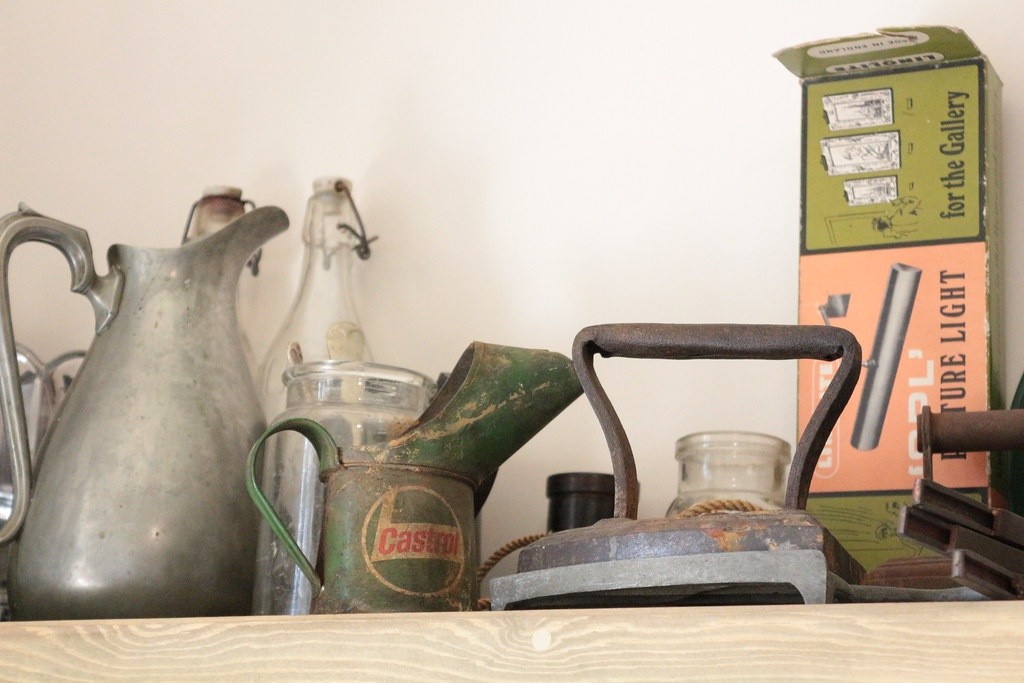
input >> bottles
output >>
[259,360,433,613]
[546,473,615,534]
[665,432,792,516]
[179,186,256,390]
[256,178,373,614]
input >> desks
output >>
[0,599,1024,683]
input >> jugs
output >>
[0,206,292,617]
[247,341,583,612]
